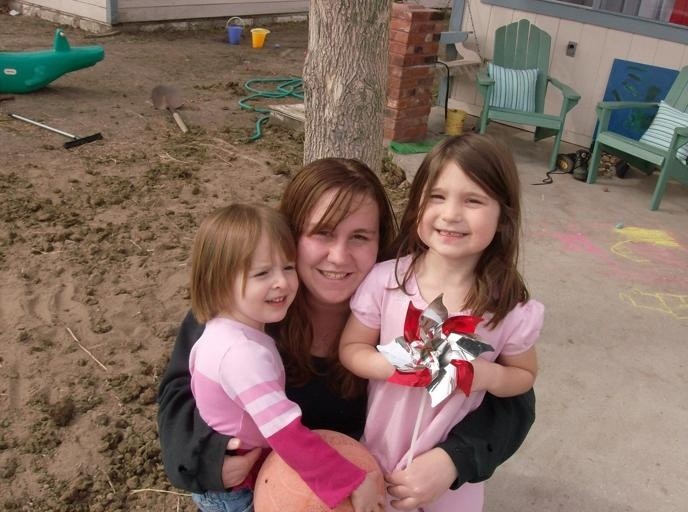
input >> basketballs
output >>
[252,429,386,512]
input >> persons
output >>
[154,157,408,510]
[335,131,546,510]
[178,200,396,510]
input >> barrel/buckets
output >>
[251,28,271,48]
[226,17,244,45]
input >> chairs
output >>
[476,20,581,170]
[586,66,688,210]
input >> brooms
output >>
[8,112,103,149]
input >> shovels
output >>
[151,85,189,134]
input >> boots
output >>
[556,152,577,172]
[572,149,591,182]
